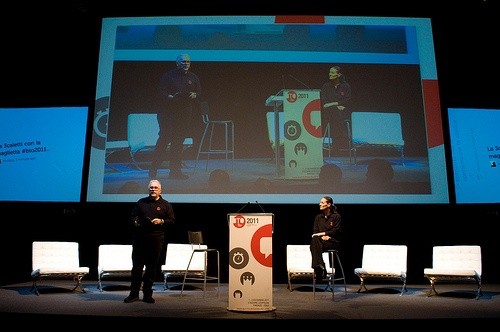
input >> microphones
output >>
[146,217,151,222]
[256,201,265,213]
[237,202,250,213]
[323,205,327,207]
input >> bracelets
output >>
[161,219,164,223]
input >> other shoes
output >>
[149,168,158,178]
[124,293,139,303]
[169,168,188,179]
[143,296,155,303]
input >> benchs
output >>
[265,111,333,170]
[160,244,209,292]
[127,113,195,171]
[285,244,336,292]
[98,245,145,292]
[30,241,90,294]
[423,245,484,302]
[354,244,409,297]
[345,111,407,168]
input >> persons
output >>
[150,53,202,180]
[122,179,173,304]
[310,197,341,278]
[321,65,351,136]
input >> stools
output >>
[195,101,235,176]
[179,231,222,303]
[312,250,348,302]
